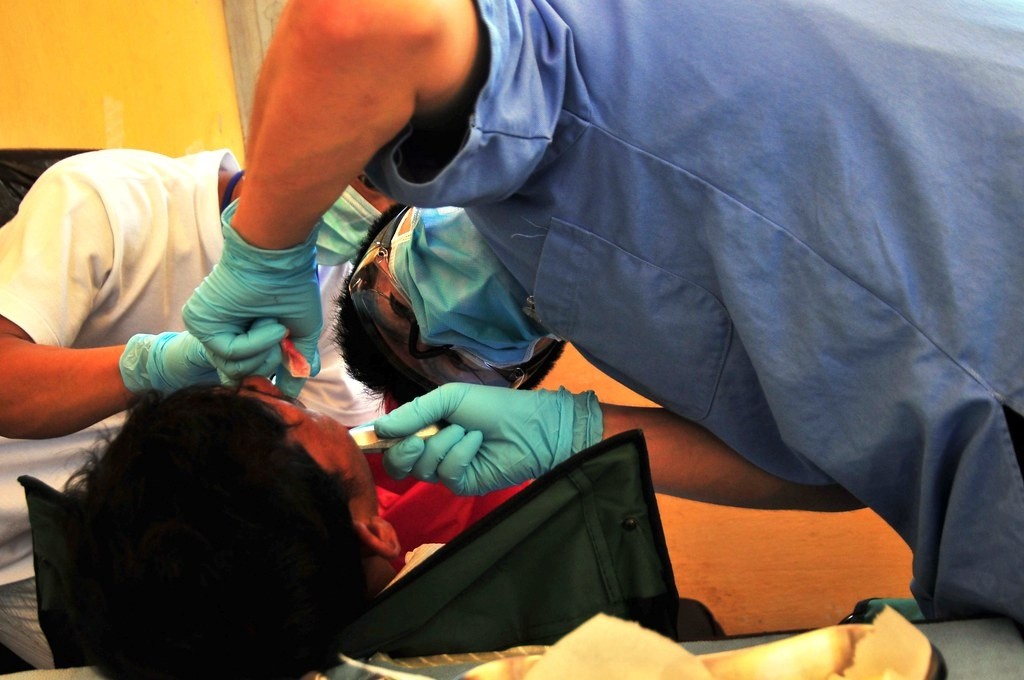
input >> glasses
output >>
[348,206,557,388]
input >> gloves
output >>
[117,319,322,401]
[182,197,324,398]
[374,381,604,498]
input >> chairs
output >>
[18,430,927,680]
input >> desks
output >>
[326,617,1024,680]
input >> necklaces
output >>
[220,168,244,213]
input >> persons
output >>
[0,0,1024,680]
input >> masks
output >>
[313,184,381,266]
[387,205,565,367]
